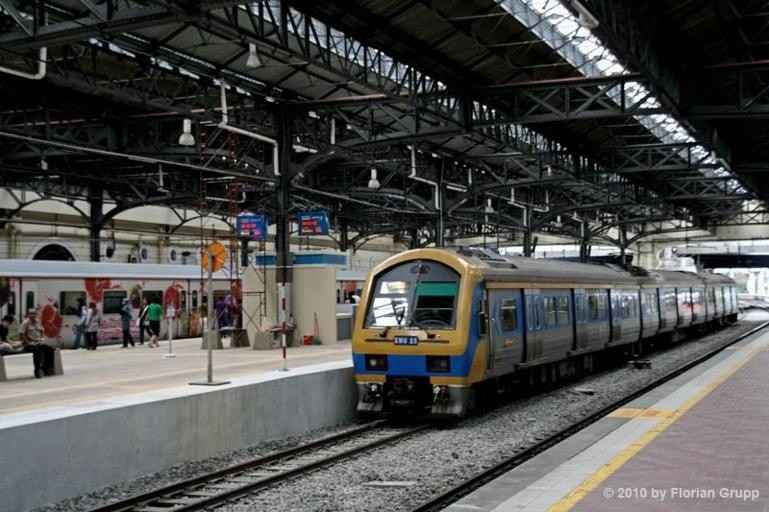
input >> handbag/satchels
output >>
[143,318,150,326]
[136,318,141,326]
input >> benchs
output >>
[0,345,63,381]
[200,329,250,350]
[252,327,298,350]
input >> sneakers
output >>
[34,370,41,378]
[41,366,49,376]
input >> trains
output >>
[350,245,740,416]
[0,256,244,356]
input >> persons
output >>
[0,290,361,378]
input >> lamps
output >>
[177,117,196,147]
[368,168,383,189]
[244,41,262,69]
